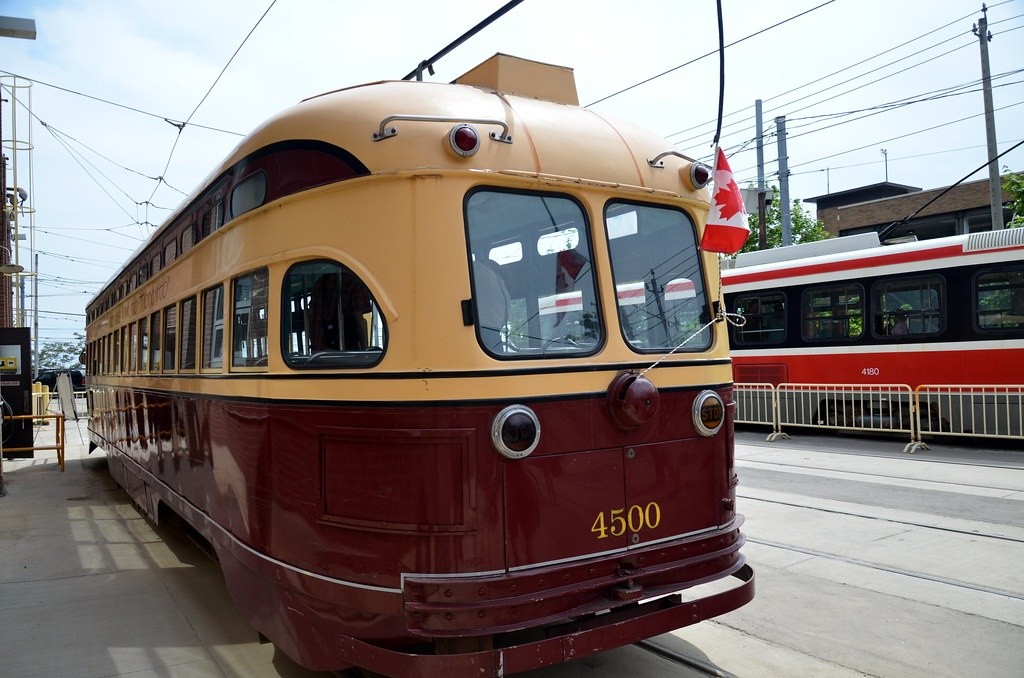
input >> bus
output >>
[79,51,755,677]
[530,228,1024,450]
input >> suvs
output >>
[33,369,86,393]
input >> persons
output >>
[471,259,511,353]
[734,298,840,344]
[893,313,909,335]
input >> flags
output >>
[697,146,750,253]
[552,247,587,328]
